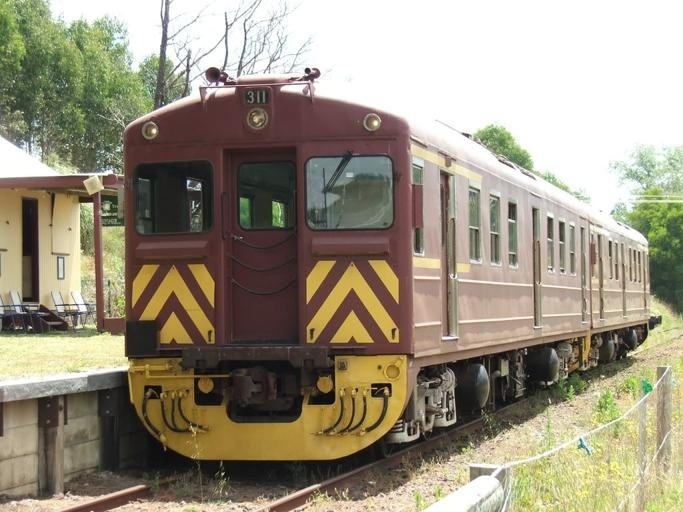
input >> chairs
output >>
[0,289,96,336]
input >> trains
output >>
[123,65,663,466]
